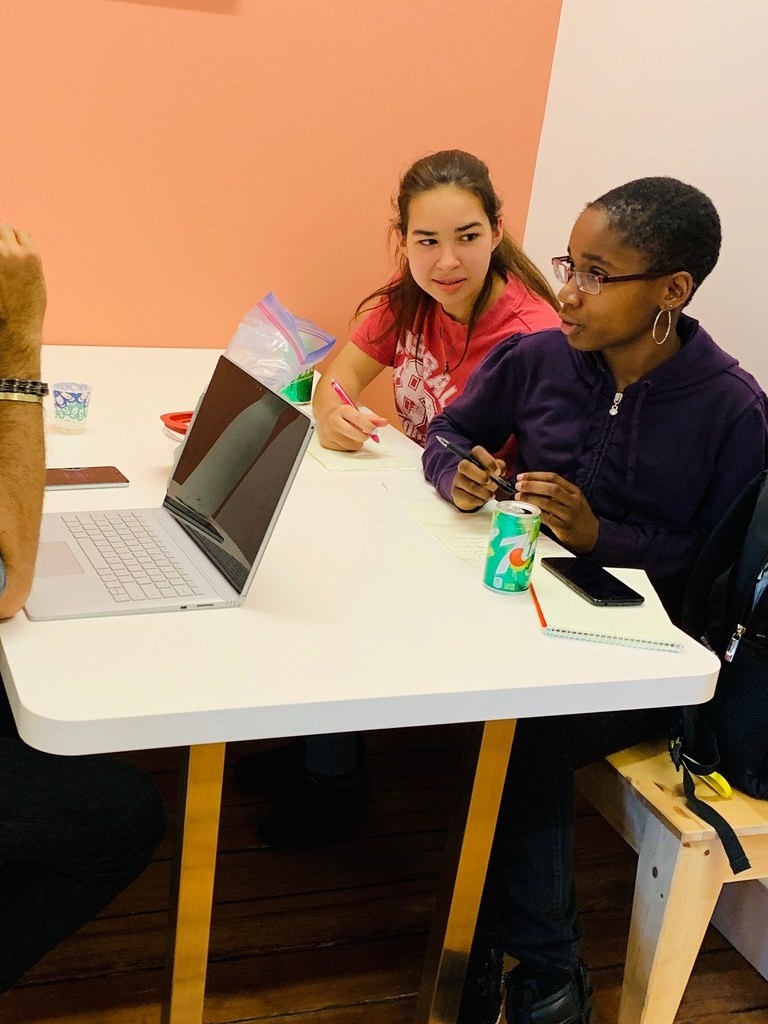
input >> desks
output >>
[0,344,722,1024]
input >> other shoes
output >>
[231,734,370,844]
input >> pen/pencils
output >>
[330,377,383,446]
[434,432,518,496]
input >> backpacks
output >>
[671,588,767,876]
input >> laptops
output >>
[23,352,319,622]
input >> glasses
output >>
[551,256,666,296]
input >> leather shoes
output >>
[457,962,594,1024]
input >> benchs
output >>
[570,743,768,1024]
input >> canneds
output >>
[482,500,541,594]
[272,332,313,404]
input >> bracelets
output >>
[5,391,44,405]
[3,377,50,396]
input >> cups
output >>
[53,382,93,431]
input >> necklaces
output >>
[436,302,467,377]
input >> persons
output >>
[417,179,766,1022]
[1,224,174,995]
[232,150,562,853]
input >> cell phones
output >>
[541,557,645,606]
[44,466,129,491]
